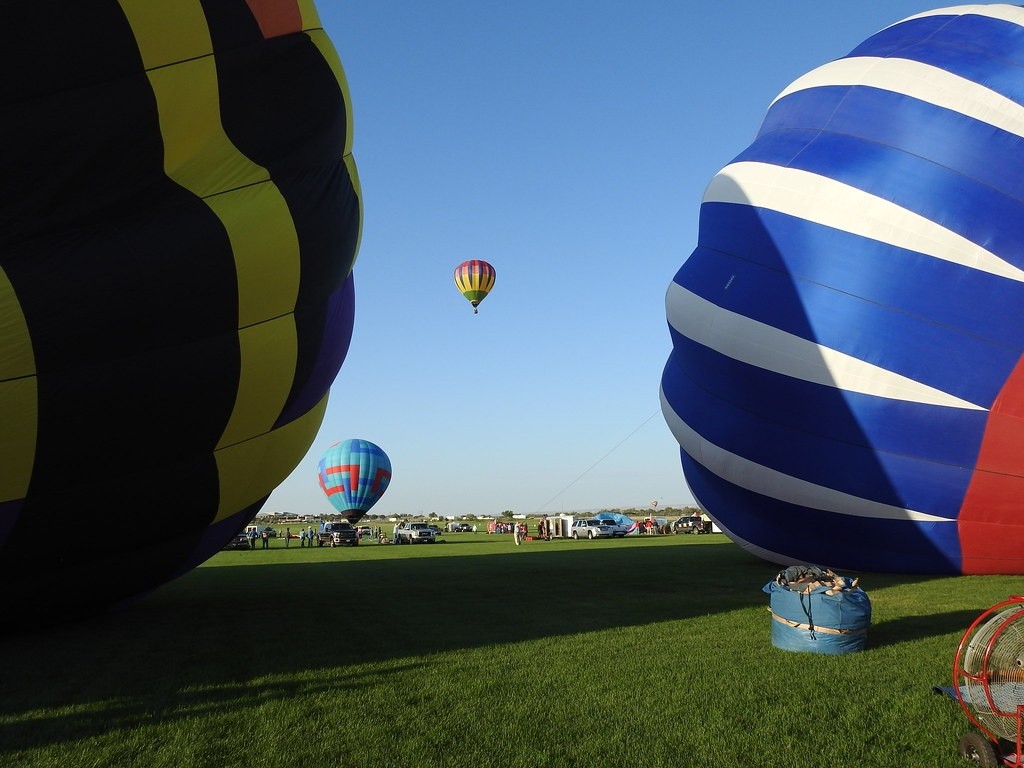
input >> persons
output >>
[248,516,660,549]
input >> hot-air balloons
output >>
[453,260,497,314]
[658,3,1024,578]
[0,0,366,648]
[316,439,392,533]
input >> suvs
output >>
[429,525,442,536]
[361,525,372,535]
[571,519,612,540]
[445,523,473,533]
[224,530,251,550]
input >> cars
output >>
[259,527,276,538]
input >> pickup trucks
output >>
[601,519,628,539]
[317,522,359,547]
[674,517,713,534]
[397,522,435,543]
